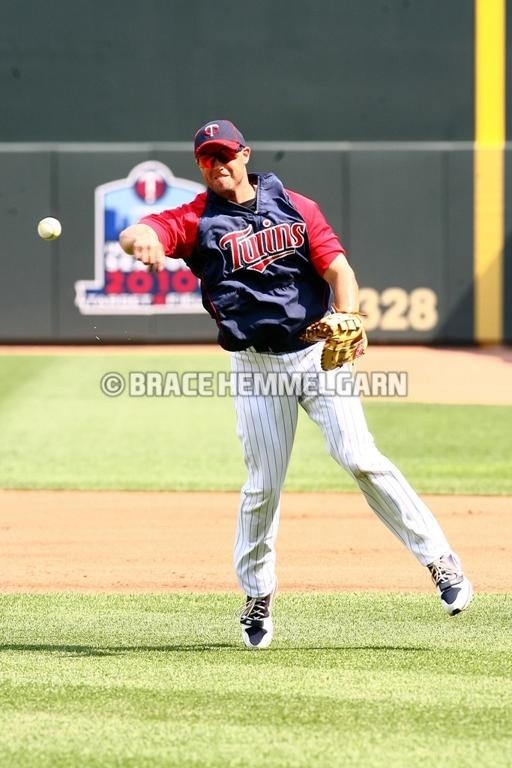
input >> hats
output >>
[194,120,246,157]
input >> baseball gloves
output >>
[300,312,367,371]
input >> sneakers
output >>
[427,551,475,616]
[234,578,280,648]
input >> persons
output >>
[114,117,478,650]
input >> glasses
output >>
[198,147,242,169]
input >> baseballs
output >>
[38,216,61,240]
